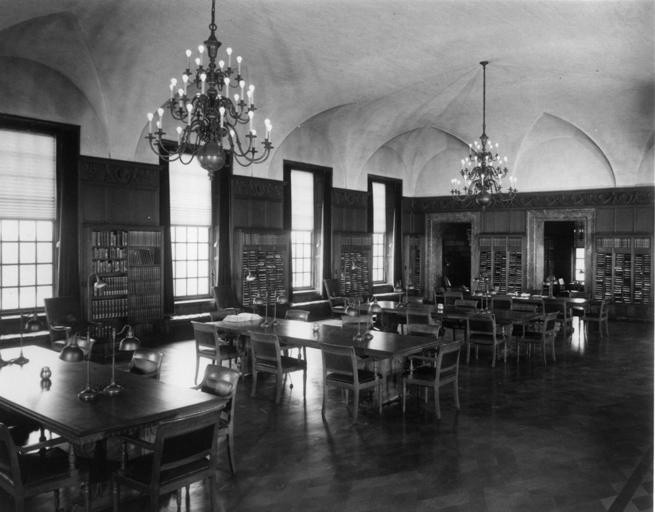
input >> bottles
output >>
[39,366,51,380]
[312,323,319,331]
[437,304,444,310]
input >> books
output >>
[242,233,286,308]
[335,236,372,296]
[91,230,161,343]
[479,237,522,293]
[591,238,652,305]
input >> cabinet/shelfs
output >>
[477,233,528,292]
[333,231,373,297]
[233,227,289,307]
[83,222,164,363]
[592,233,654,322]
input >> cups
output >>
[516,291,521,296]
[491,292,496,297]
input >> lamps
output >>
[450,61,517,211]
[144,0,273,180]
